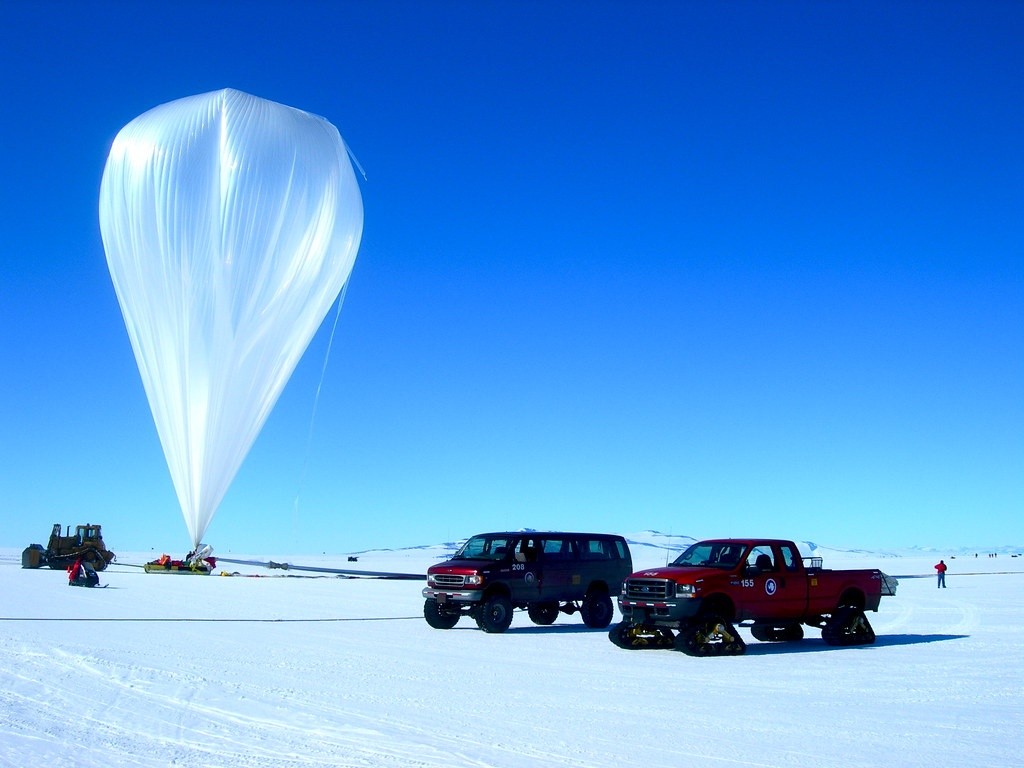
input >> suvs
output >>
[420,530,634,634]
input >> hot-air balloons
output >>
[97,87,365,578]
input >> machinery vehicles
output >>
[21,523,115,572]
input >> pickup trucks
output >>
[607,538,897,659]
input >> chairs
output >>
[755,554,774,569]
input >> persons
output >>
[934,560,947,588]
[975,553,997,558]
[68,557,82,586]
